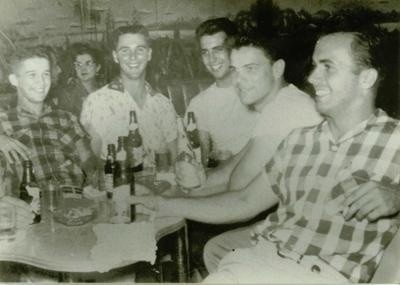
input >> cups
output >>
[0,201,18,242]
[44,180,60,212]
[155,148,168,173]
[213,151,232,164]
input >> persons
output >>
[175,32,324,196]
[54,42,101,117]
[185,18,258,164]
[112,22,399,284]
[79,25,177,188]
[0,48,98,210]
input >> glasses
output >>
[73,61,96,67]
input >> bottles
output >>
[105,144,116,198]
[175,116,203,189]
[127,112,143,171]
[185,113,203,165]
[20,161,41,224]
[114,136,137,222]
